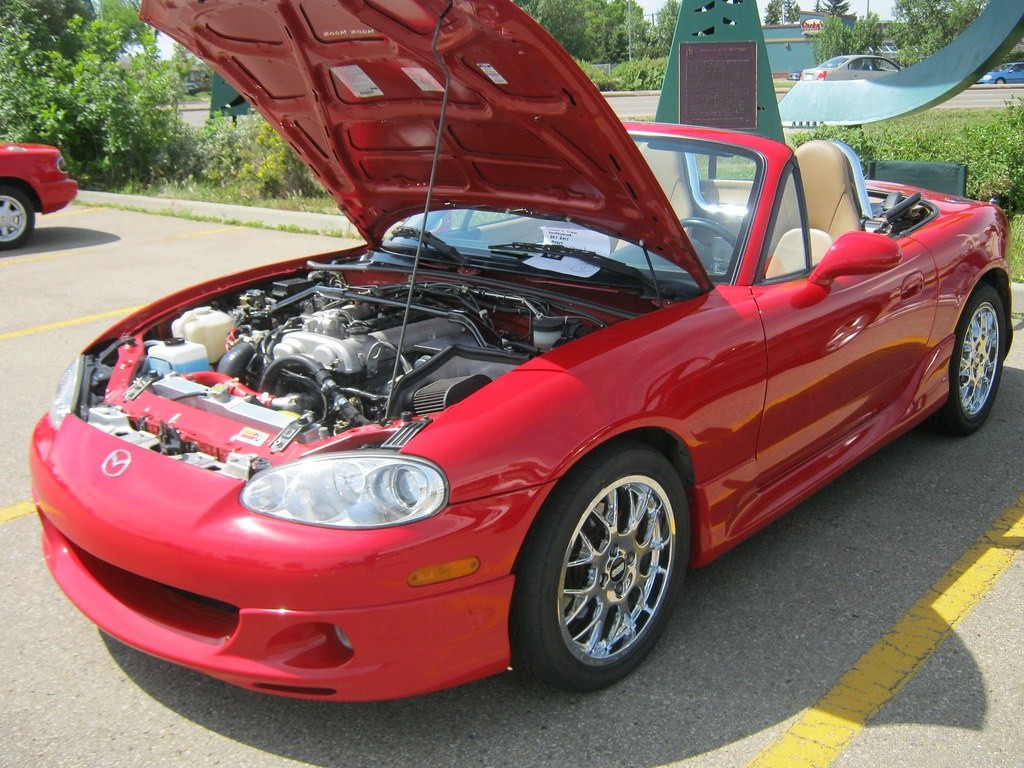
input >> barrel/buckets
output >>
[148,337,210,377]
[170,305,236,368]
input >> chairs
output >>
[754,138,875,288]
[613,139,712,256]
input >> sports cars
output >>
[29,0,1018,704]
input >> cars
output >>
[786,71,801,81]
[802,54,903,81]
[0,141,79,251]
[976,62,1024,84]
